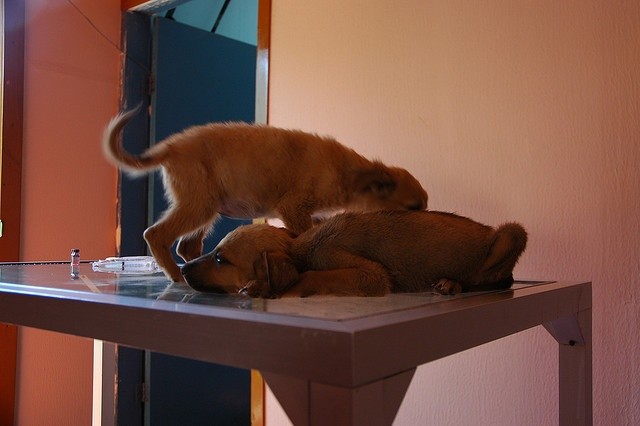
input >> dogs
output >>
[100,98,431,284]
[180,206,527,302]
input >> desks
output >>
[1,257,592,426]
